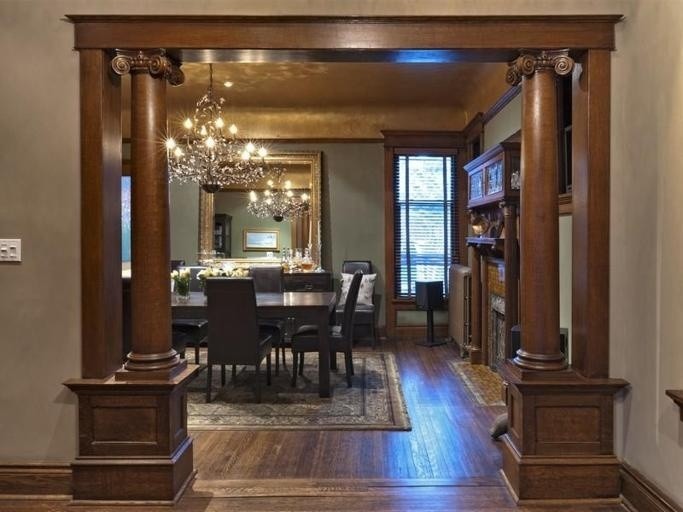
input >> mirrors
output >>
[196,149,322,273]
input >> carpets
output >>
[175,348,413,431]
[451,360,508,406]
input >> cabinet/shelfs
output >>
[463,143,520,369]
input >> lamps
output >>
[161,63,269,194]
[415,280,443,347]
[246,164,312,222]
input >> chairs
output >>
[121,263,365,397]
[342,260,377,346]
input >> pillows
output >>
[337,272,377,306]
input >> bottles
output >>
[280,247,315,271]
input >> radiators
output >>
[447,264,473,358]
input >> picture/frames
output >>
[242,227,280,252]
